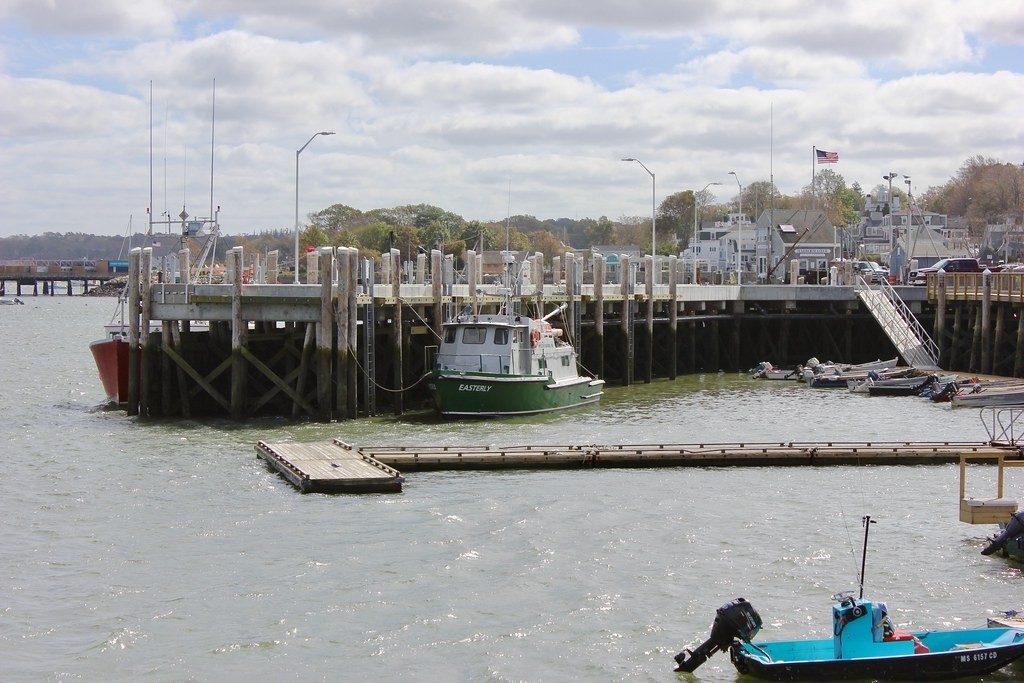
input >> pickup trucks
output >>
[908,257,1005,287]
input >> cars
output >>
[849,260,887,285]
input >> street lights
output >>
[620,157,656,284]
[883,172,897,254]
[692,182,723,282]
[728,171,742,285]
[291,130,337,285]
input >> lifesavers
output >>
[530,328,539,348]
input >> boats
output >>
[758,356,1024,407]
[89,215,210,405]
[422,179,607,416]
[729,515,1024,683]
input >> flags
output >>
[816,149,838,164]
